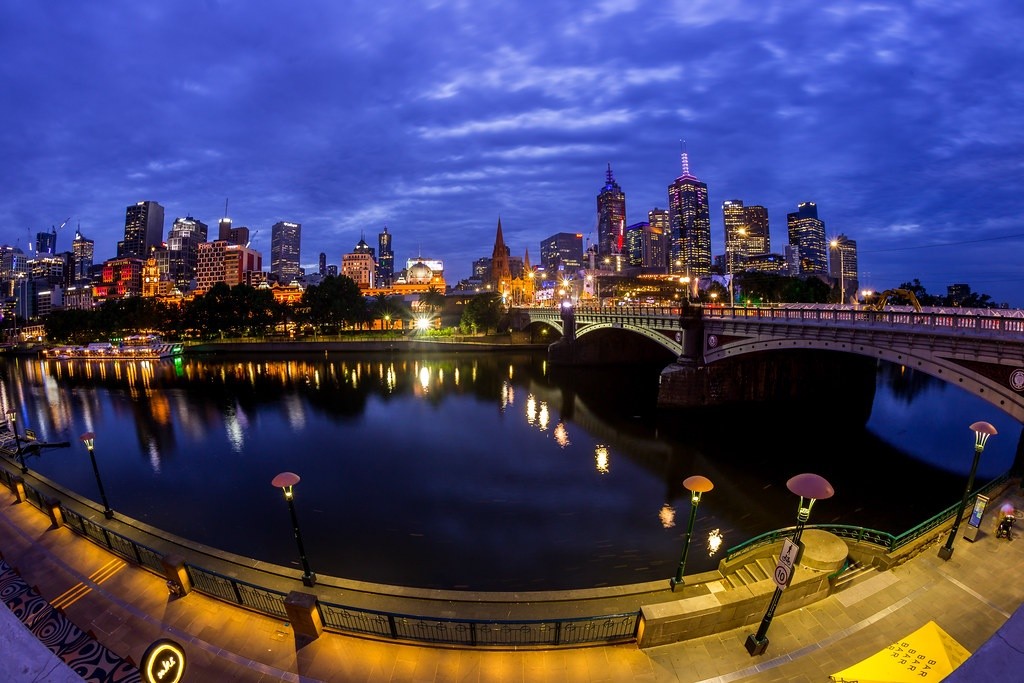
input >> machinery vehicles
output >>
[863,287,926,325]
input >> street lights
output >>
[945,421,998,550]
[6,408,26,468]
[830,238,844,305]
[81,431,110,512]
[754,473,835,641]
[272,472,311,577]
[675,258,689,302]
[673,475,713,582]
[728,226,749,306]
